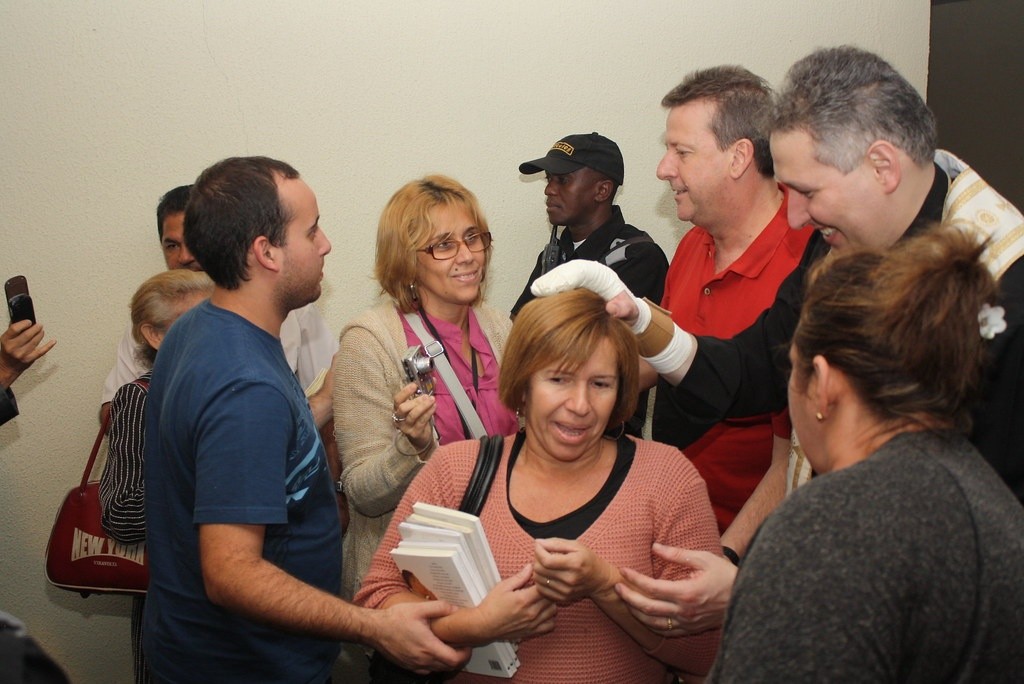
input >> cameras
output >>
[401,345,435,398]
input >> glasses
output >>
[416,231,491,260]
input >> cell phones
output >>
[5,276,36,330]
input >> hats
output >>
[519,132,624,186]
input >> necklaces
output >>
[418,306,479,440]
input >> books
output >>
[391,502,521,678]
[304,367,329,398]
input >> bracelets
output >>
[723,546,739,566]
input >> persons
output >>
[93,268,219,545]
[136,155,473,684]
[101,186,350,531]
[350,286,721,684]
[0,320,56,426]
[631,62,813,567]
[507,131,670,442]
[402,570,437,601]
[530,44,1024,639]
[704,218,1024,684]
[332,173,526,667]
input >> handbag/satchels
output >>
[45,380,151,597]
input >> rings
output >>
[392,412,405,422]
[667,616,673,631]
[546,579,550,587]
